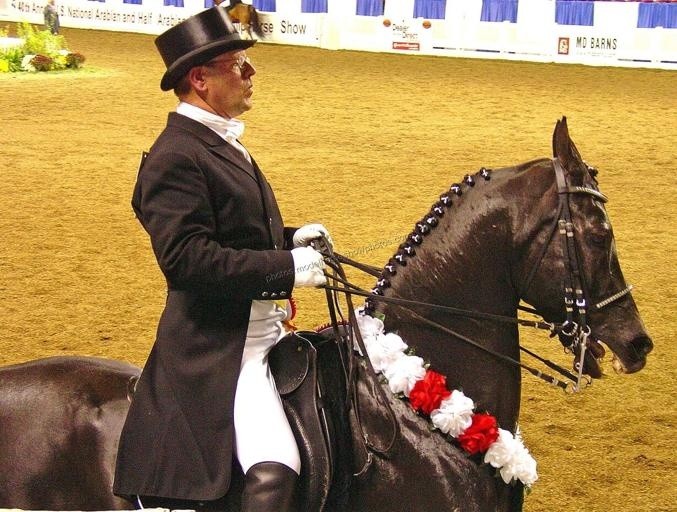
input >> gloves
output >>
[290,247,329,289]
[293,223,333,249]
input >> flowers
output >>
[63,52,85,66]
[30,55,54,65]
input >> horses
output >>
[212,0,264,41]
[0,115,655,512]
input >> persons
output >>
[220,0,242,11]
[44,0,59,35]
[112,5,333,512]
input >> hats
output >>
[154,6,257,92]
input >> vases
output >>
[66,65,78,68]
[35,66,49,71]
